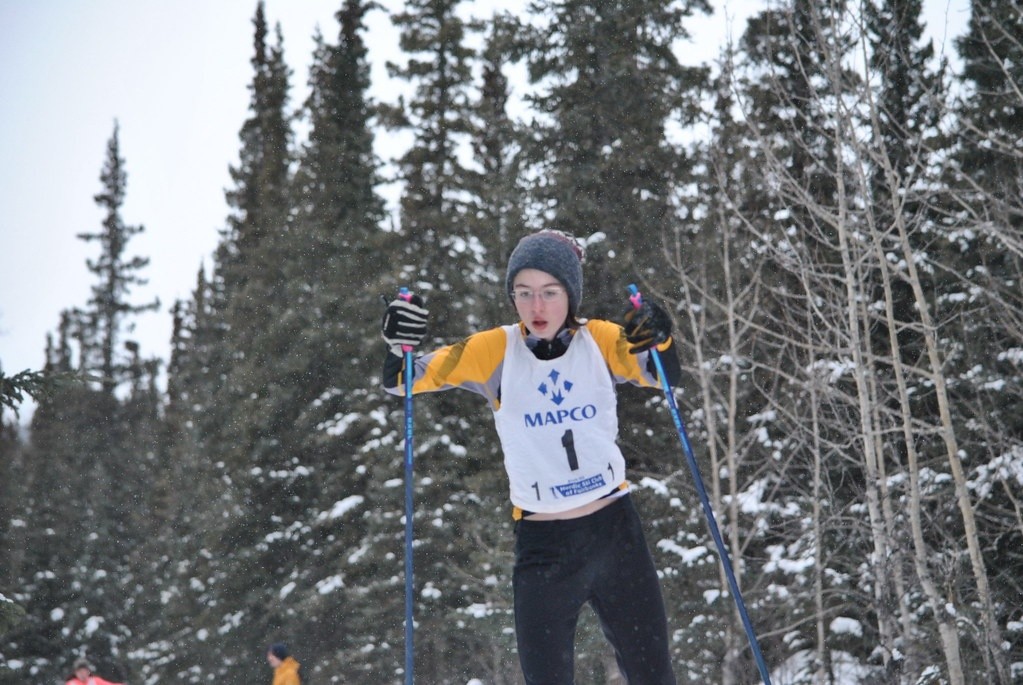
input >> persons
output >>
[382,230,681,685]
[65,657,127,685]
[267,643,304,685]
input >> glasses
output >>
[510,287,565,303]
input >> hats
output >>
[271,643,289,660]
[505,228,587,316]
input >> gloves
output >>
[623,298,671,355]
[380,295,429,357]
